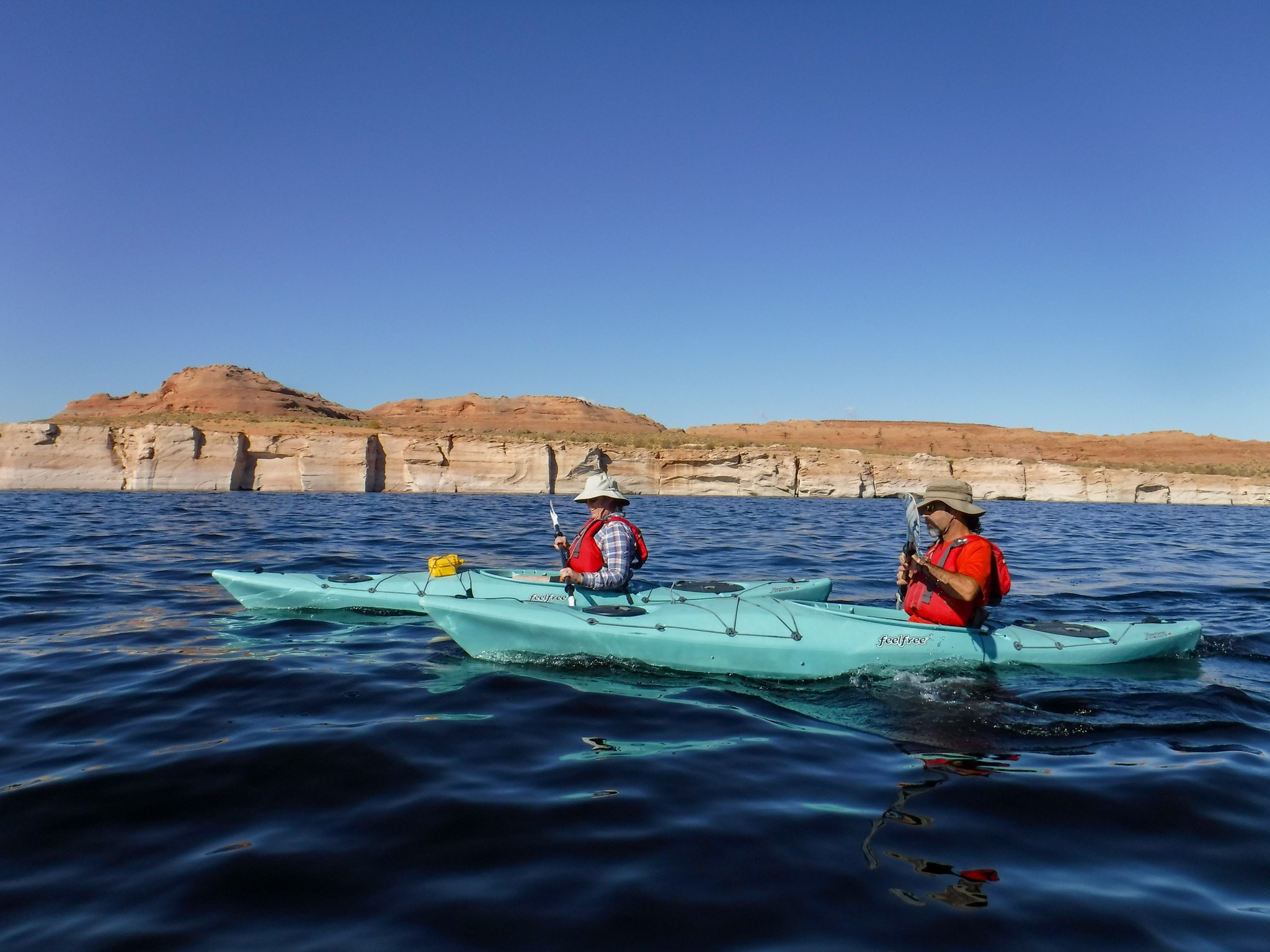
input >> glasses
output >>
[583,497,606,503]
[926,503,953,512]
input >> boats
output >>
[210,550,833,627]
[415,550,1206,682]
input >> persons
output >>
[896,479,992,629]
[511,474,637,590]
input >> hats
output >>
[572,473,630,506]
[915,479,987,517]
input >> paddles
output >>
[894,493,920,617]
[541,447,573,595]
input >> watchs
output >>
[916,557,931,573]
